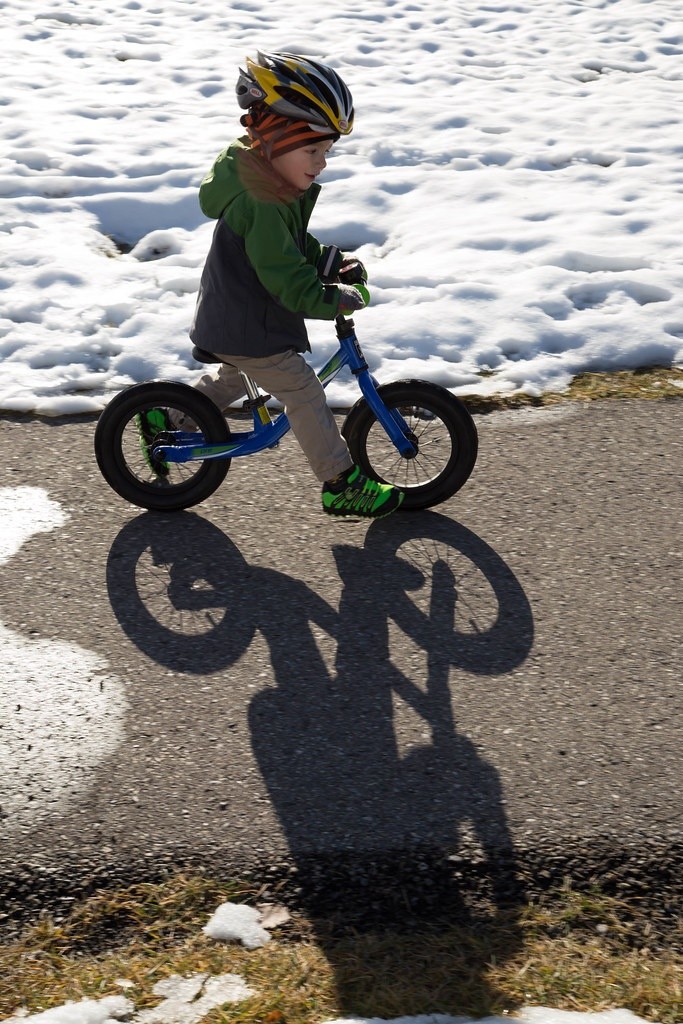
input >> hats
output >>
[239,103,341,159]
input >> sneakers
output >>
[321,466,404,519]
[135,407,174,478]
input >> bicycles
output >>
[93,255,480,512]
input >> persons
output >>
[134,50,405,520]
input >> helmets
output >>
[235,48,356,134]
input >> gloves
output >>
[331,281,365,310]
[337,258,366,286]
